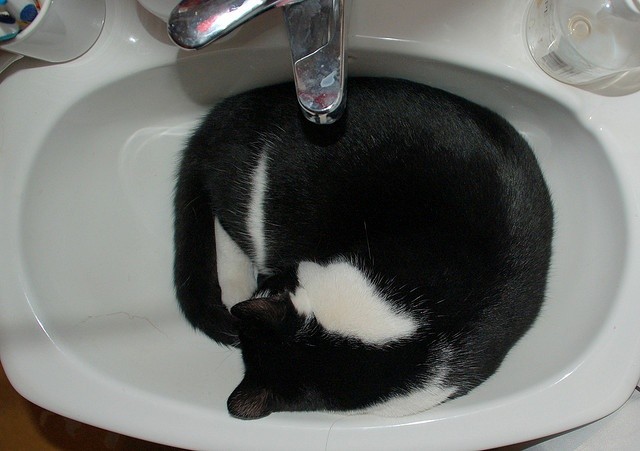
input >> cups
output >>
[0,0,111,68]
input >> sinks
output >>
[20,47,629,429]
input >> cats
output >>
[171,75,555,423]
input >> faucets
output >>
[166,1,353,126]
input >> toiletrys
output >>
[525,1,640,85]
